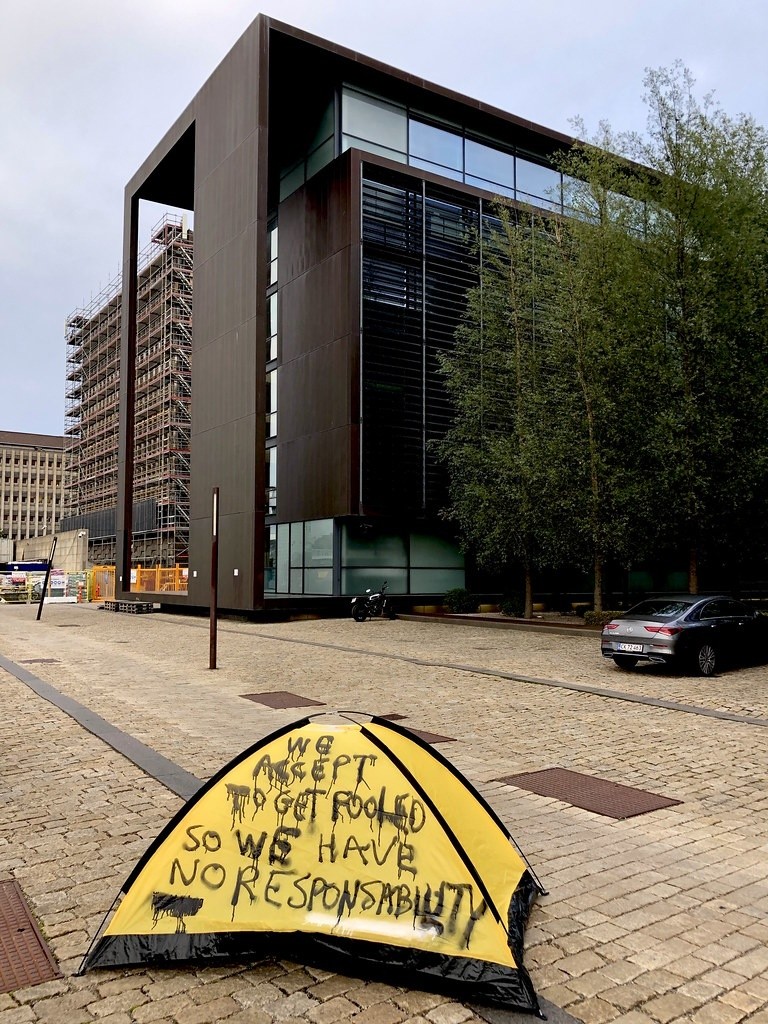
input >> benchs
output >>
[104,599,154,614]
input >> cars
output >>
[601,592,768,677]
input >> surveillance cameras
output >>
[78,533,82,538]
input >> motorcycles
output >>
[350,581,396,622]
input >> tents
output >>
[77,711,551,1023]
